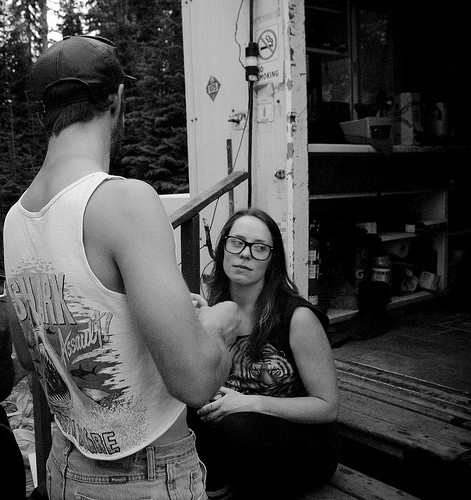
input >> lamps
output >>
[245,43,262,81]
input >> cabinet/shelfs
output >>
[306,46,452,326]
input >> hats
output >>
[28,34,138,113]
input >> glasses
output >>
[223,235,275,261]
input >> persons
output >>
[3,33,243,500]
[186,207,340,500]
